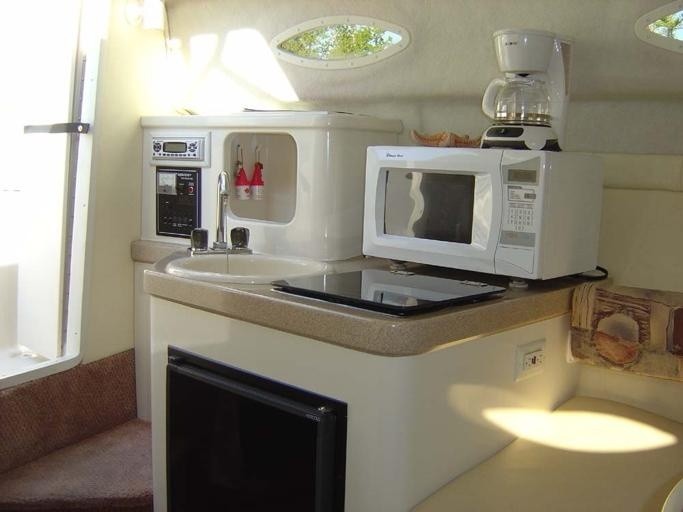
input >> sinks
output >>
[164,253,329,287]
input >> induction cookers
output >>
[270,264,510,318]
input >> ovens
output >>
[167,341,348,512]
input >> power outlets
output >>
[514,338,545,380]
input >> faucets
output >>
[211,171,228,250]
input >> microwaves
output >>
[360,138,607,287]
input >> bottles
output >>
[250,165,265,201]
[234,167,250,202]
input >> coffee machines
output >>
[477,24,576,152]
